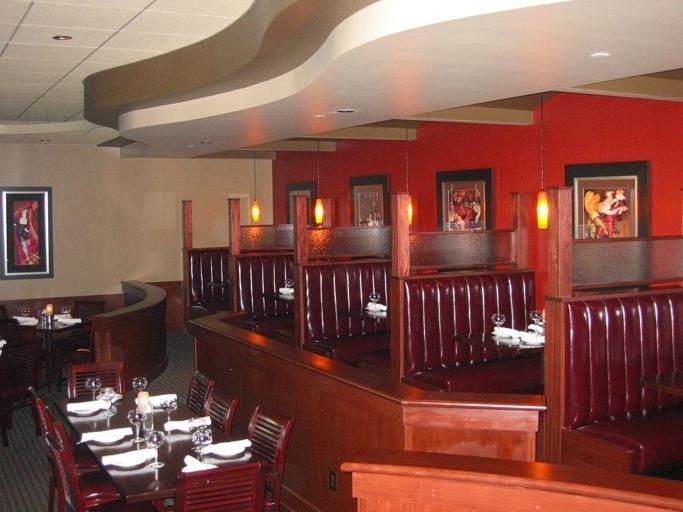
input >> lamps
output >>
[406,121,413,227]
[536,92,548,229]
[315,139,324,224]
[251,151,260,222]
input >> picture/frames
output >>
[350,175,391,260]
[286,182,316,225]
[0,186,54,280]
[564,160,649,292]
[436,167,496,271]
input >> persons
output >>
[596,188,628,242]
[12,209,32,264]
[589,190,628,235]
[446,185,483,232]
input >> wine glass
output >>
[369,292,381,312]
[132,377,148,392]
[19,304,72,328]
[284,279,294,296]
[128,407,153,443]
[100,387,116,415]
[144,430,167,468]
[85,377,101,401]
[188,417,213,466]
[491,309,545,345]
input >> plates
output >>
[212,442,245,457]
[93,432,125,444]
[113,453,146,469]
[134,395,173,408]
[11,316,76,327]
[70,394,123,416]
